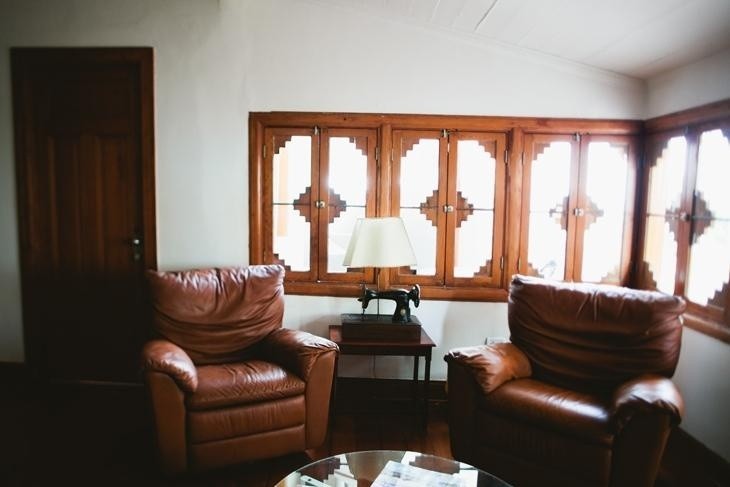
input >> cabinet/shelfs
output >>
[9,45,157,388]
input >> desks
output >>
[330,325,436,433]
[272,449,513,487]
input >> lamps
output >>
[344,216,415,316]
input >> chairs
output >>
[140,263,341,480]
[443,273,688,487]
